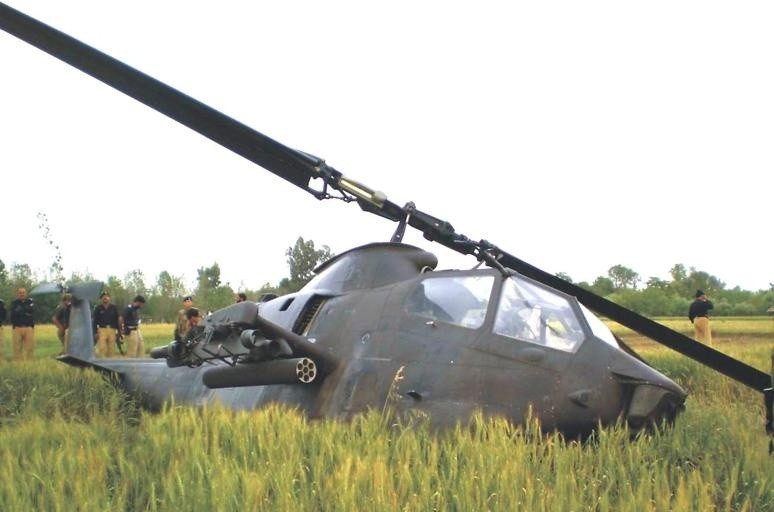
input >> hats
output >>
[184,297,190,301]
[99,291,109,299]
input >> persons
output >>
[173,296,202,342]
[51,293,71,355]
[117,295,145,357]
[183,307,201,334]
[236,293,246,304]
[93,291,119,357]
[0,299,8,328]
[9,286,35,361]
[688,289,714,347]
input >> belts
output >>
[99,325,114,329]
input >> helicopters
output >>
[0,1,774,450]
